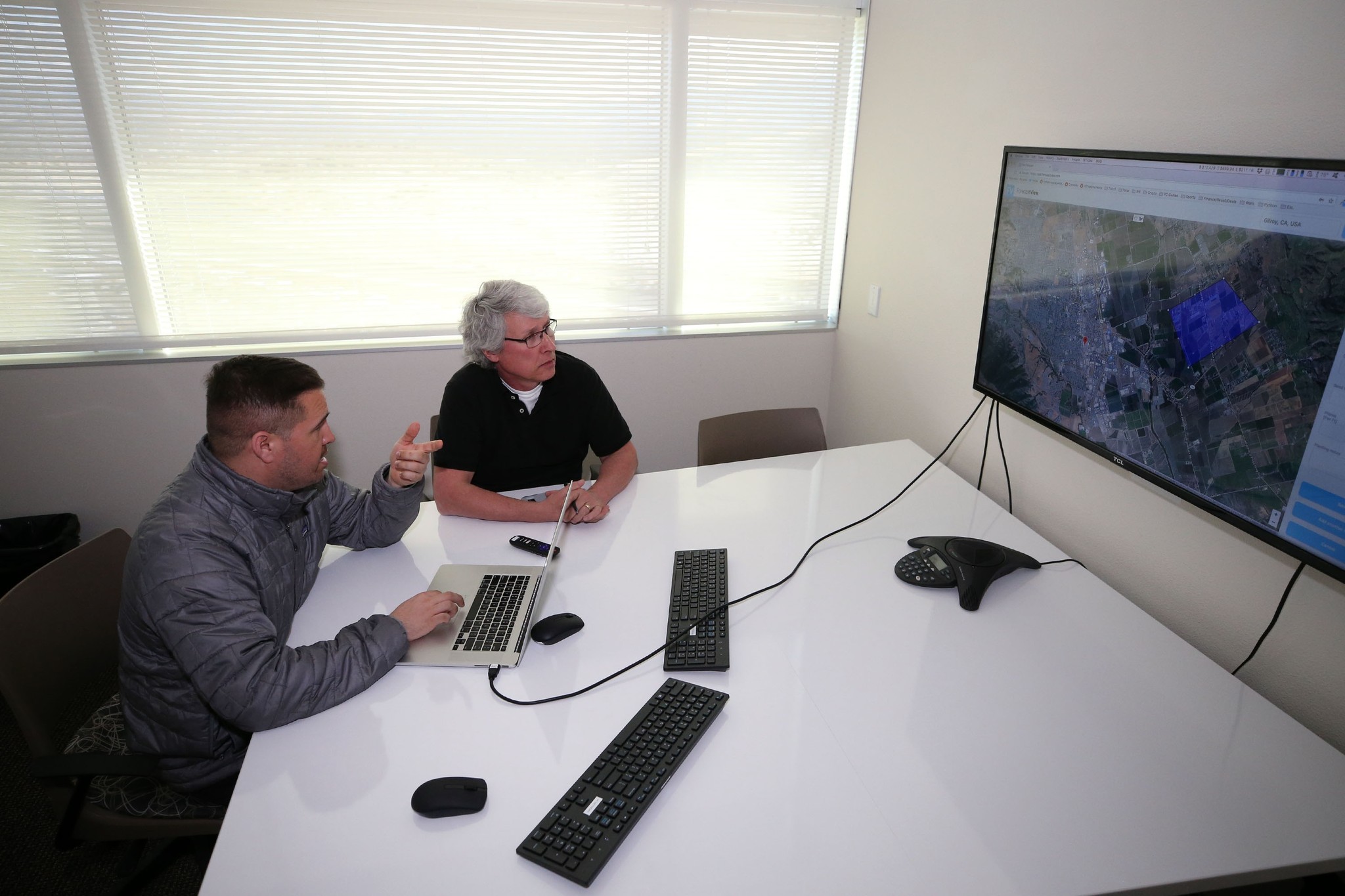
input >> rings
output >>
[585,504,591,512]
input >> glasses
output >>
[505,319,560,349]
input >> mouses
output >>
[410,776,488,819]
[529,612,585,646]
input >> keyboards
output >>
[514,678,731,888]
[663,548,730,672]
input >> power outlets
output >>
[867,284,880,317]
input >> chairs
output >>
[0,529,227,896]
[0,513,81,599]
[697,407,827,467]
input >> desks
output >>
[197,440,1345,896]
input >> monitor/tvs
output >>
[972,145,1345,585]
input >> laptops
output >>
[393,479,573,668]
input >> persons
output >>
[115,355,465,806]
[433,280,638,525]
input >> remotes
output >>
[509,534,560,559]
